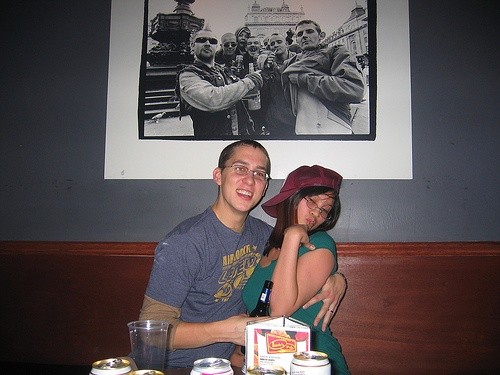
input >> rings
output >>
[328,309,333,313]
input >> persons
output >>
[128,140,351,375]
[175,20,367,136]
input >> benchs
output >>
[0,240,499,373]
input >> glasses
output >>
[303,197,332,219]
[224,44,236,47]
[224,164,270,182]
[194,37,218,44]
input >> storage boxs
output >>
[244,314,310,374]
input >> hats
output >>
[261,165,343,218]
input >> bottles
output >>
[240,280,273,354]
[247,63,261,112]
[234,55,244,79]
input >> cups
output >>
[126,320,169,371]
